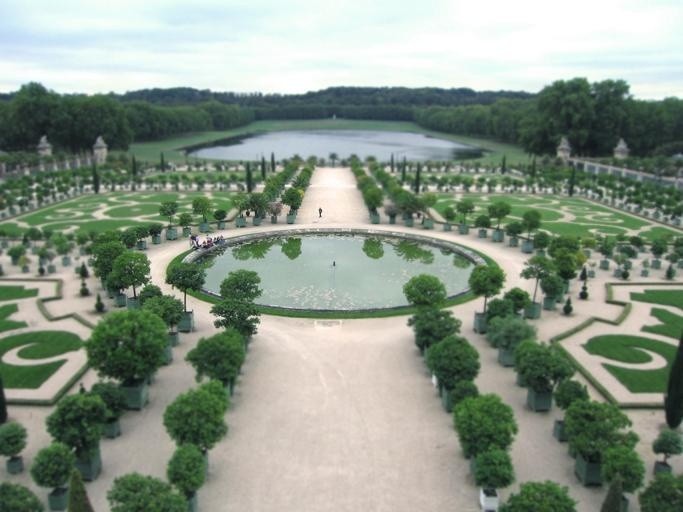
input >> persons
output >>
[188,232,224,250]
[319,207,322,217]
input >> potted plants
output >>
[346,153,681,512]
[0,150,315,511]
